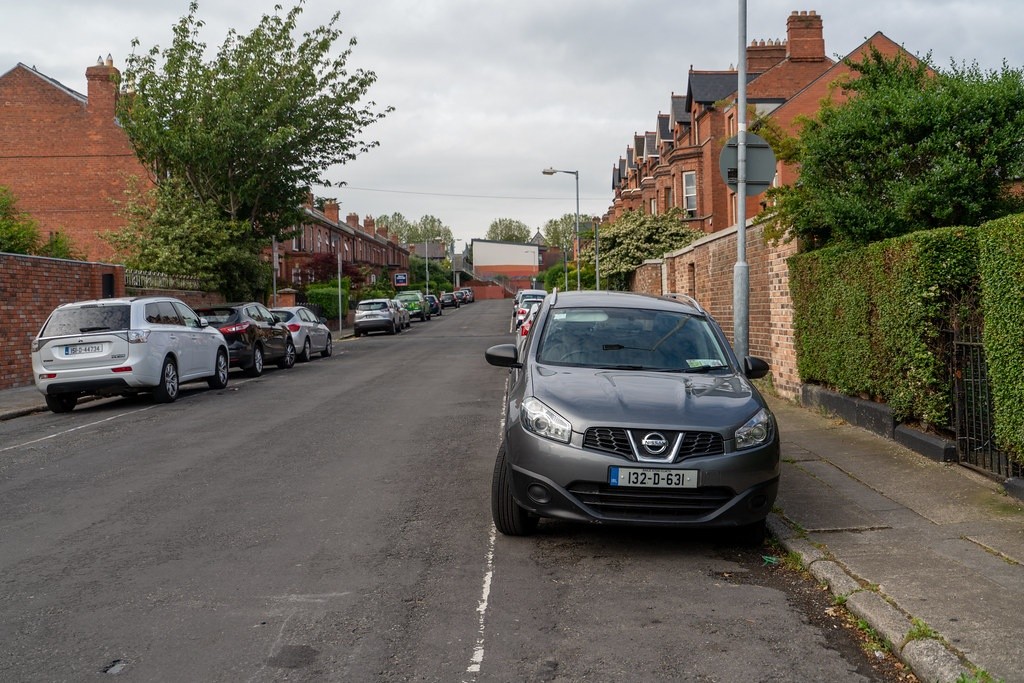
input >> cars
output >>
[268,305,333,362]
[513,288,548,357]
[371,299,410,329]
[393,289,431,322]
[440,287,474,309]
[424,294,444,316]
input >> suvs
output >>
[483,286,782,550]
[353,299,402,338]
[30,296,230,414]
[191,301,296,378]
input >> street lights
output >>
[425,236,443,295]
[452,238,463,288]
[542,167,581,291]
